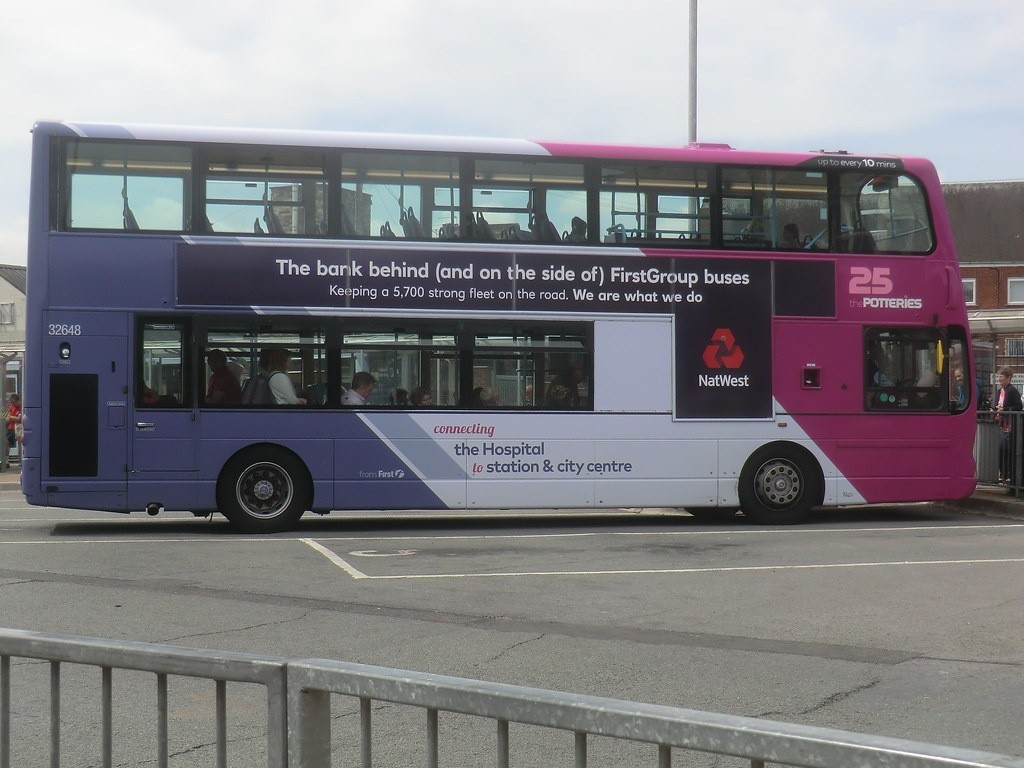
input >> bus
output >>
[14,118,982,537]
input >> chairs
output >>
[476,211,495,240]
[385,221,396,237]
[123,208,140,230]
[530,215,537,241]
[320,220,328,236]
[509,226,521,240]
[263,205,285,234]
[472,213,481,241]
[342,205,357,236]
[852,215,877,253]
[240,377,252,397]
[380,225,386,238]
[631,228,639,237]
[253,218,264,234]
[544,211,561,243]
[446,223,457,238]
[399,211,413,237]
[408,207,424,238]
[439,227,447,238]
[841,224,850,250]
[501,230,509,240]
[803,235,817,250]
[249,374,268,405]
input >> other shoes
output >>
[998,478,1010,484]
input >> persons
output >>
[545,360,585,408]
[259,348,307,404]
[6,394,22,468]
[783,224,800,245]
[865,339,897,407]
[139,368,178,408]
[526,385,532,404]
[955,369,982,410]
[566,217,587,240]
[989,366,1023,482]
[340,372,375,405]
[747,222,764,240]
[390,388,432,405]
[205,350,232,401]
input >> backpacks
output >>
[251,372,286,406]
[976,382,990,419]
